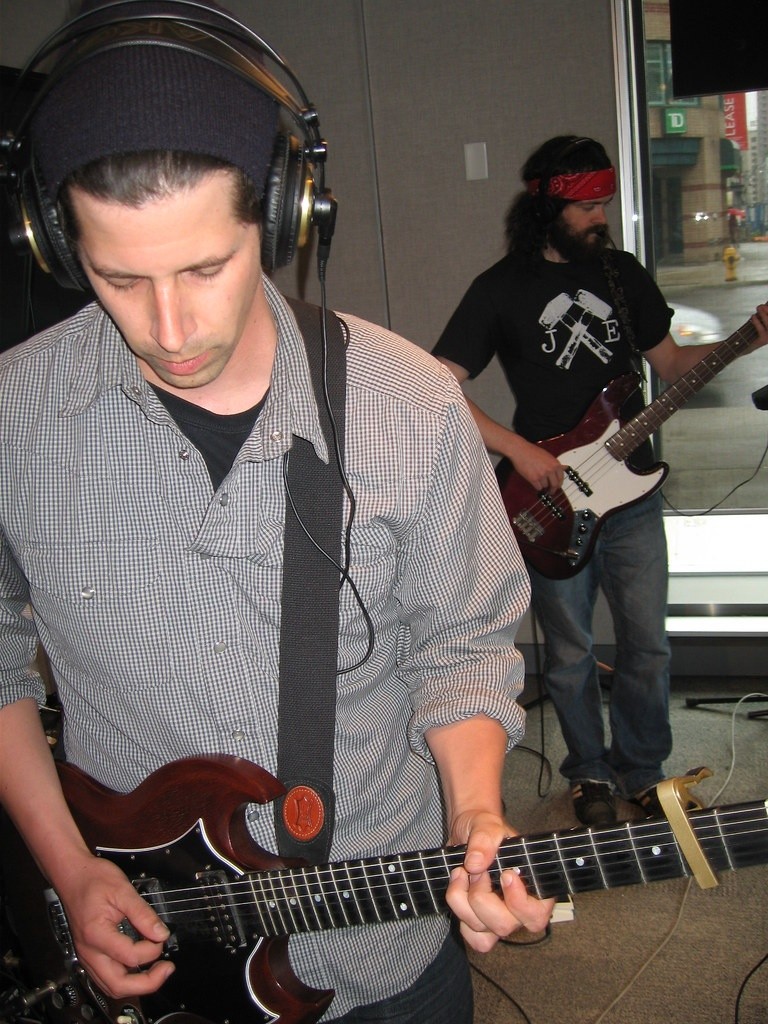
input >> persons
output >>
[431,135,768,829]
[2,1,558,1024]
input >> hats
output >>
[32,0,284,202]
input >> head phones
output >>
[0,0,338,294]
[528,137,592,225]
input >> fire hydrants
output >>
[722,248,741,281]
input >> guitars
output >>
[0,751,768,1024]
[496,304,768,580]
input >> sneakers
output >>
[569,780,617,826]
[619,786,698,819]
